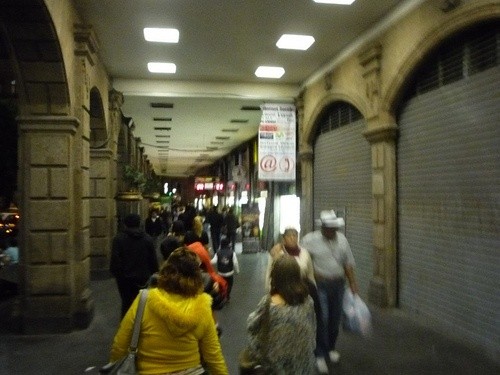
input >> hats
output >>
[314,209,344,227]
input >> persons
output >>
[142,195,242,338]
[299,209,358,373]
[108,213,158,322]
[264,227,317,297]
[105,246,229,375]
[238,254,318,375]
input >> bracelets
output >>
[347,274,357,280]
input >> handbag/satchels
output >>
[237,346,273,375]
[99,351,136,375]
[341,287,373,338]
